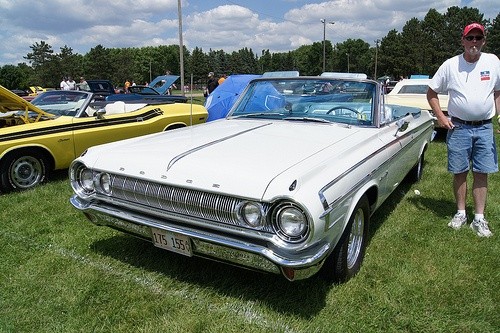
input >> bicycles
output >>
[0,74,209,194]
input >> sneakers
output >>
[447,213,467,230]
[470,218,492,237]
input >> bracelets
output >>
[496,115,500,120]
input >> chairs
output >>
[91,83,100,90]
[74,99,97,116]
[358,105,394,122]
[102,83,108,90]
[104,100,125,116]
[312,109,336,116]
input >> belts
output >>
[452,117,492,125]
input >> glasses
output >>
[465,35,484,41]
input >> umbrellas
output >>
[204,75,286,122]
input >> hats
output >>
[63,76,68,80]
[463,22,484,39]
[80,77,85,79]
[208,72,214,77]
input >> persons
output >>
[426,24,500,237]
[79,77,88,91]
[162,69,177,95]
[322,82,332,93]
[146,81,148,86]
[60,75,76,90]
[206,71,228,96]
[124,79,137,94]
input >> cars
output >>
[68,70,450,286]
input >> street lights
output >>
[319,18,335,72]
[374,38,381,81]
